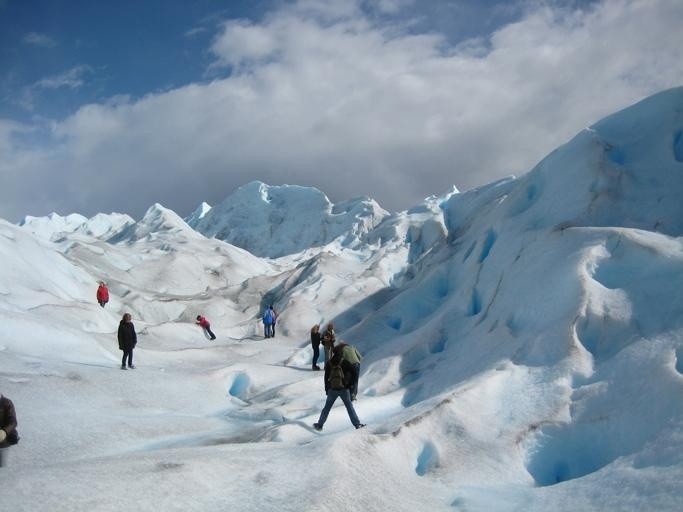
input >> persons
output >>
[195,315,215,340]
[321,323,336,370]
[312,346,365,430]
[338,339,362,401]
[262,304,274,338]
[96,282,109,307]
[0,392,18,470]
[118,312,137,370]
[269,305,278,338]
[310,324,319,370]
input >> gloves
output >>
[0,429,7,443]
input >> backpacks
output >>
[328,358,345,390]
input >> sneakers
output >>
[313,423,322,430]
[351,393,356,401]
[264,335,275,337]
[122,365,136,370]
[312,365,319,370]
[355,423,366,428]
[211,336,216,340]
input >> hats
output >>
[333,341,348,353]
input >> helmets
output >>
[124,314,131,320]
